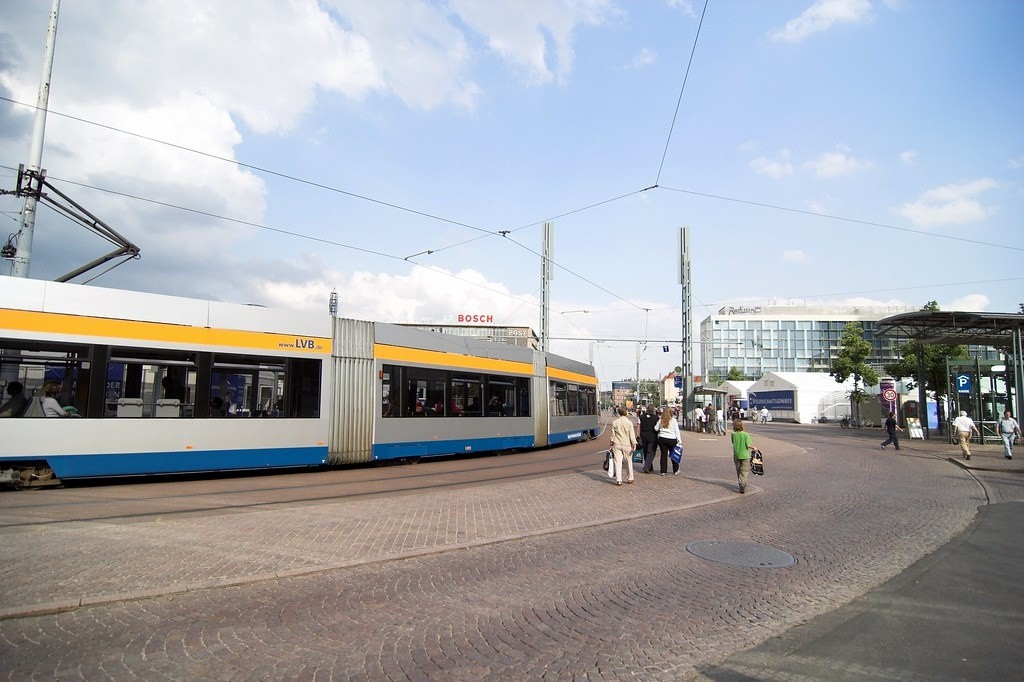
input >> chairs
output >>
[23,392,45,418]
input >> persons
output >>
[996,410,1022,460]
[751,406,758,423]
[880,412,903,451]
[606,403,682,485]
[696,403,726,436]
[952,410,980,461]
[41,379,80,418]
[211,396,225,416]
[731,420,758,493]
[727,402,745,420]
[633,402,680,417]
[0,381,28,418]
[162,376,173,399]
[760,406,768,424]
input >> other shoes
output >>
[737,483,745,494]
[880,443,886,450]
[628,480,633,483]
[643,467,650,474]
[895,448,900,450]
[616,482,622,486]
[963,452,967,458]
[661,472,668,476]
[674,469,680,475]
[966,455,971,460]
[1005,456,1013,460]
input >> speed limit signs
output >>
[884,390,897,401]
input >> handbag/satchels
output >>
[632,438,643,464]
[952,438,959,445]
[603,450,615,478]
[750,450,764,475]
[999,421,1002,434]
[669,444,683,463]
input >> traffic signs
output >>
[674,376,682,388]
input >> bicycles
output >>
[840,414,873,429]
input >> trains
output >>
[2,274,601,487]
